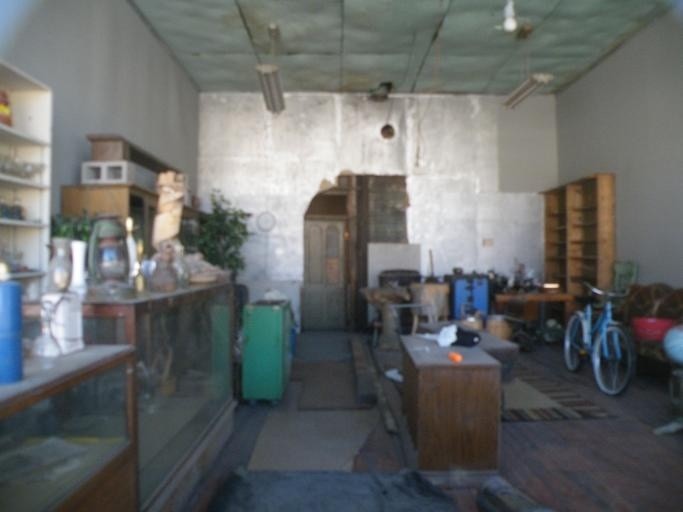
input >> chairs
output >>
[620,282,683,376]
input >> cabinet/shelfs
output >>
[537,172,614,330]
[1,336,141,510]
[23,279,244,511]
[2,57,54,303]
[59,184,214,259]
[244,299,291,403]
[398,334,505,476]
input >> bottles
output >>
[37,236,87,358]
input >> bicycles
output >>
[564,276,636,397]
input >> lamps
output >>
[499,22,556,110]
[255,24,287,114]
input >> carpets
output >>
[493,346,621,422]
